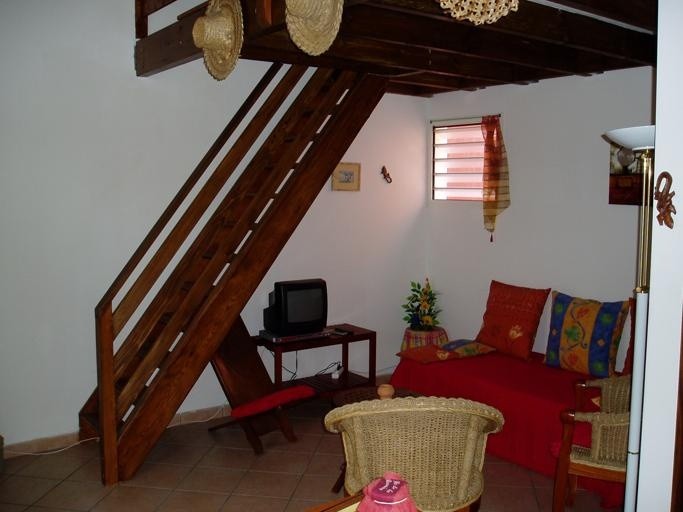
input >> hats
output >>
[285,0,344,58]
[193,0,244,81]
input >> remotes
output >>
[335,327,353,334]
[330,331,348,336]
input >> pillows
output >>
[440,339,496,358]
[542,288,631,380]
[470,278,553,362]
[396,345,461,367]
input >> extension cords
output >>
[332,367,346,378]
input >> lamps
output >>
[604,123,655,512]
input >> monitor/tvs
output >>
[262,278,328,337]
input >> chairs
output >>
[323,393,507,510]
[550,376,630,511]
[207,314,316,455]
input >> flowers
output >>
[403,277,444,327]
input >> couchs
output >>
[394,297,626,508]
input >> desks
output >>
[333,385,419,495]
[401,325,448,352]
[251,321,376,388]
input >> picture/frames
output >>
[331,162,362,193]
